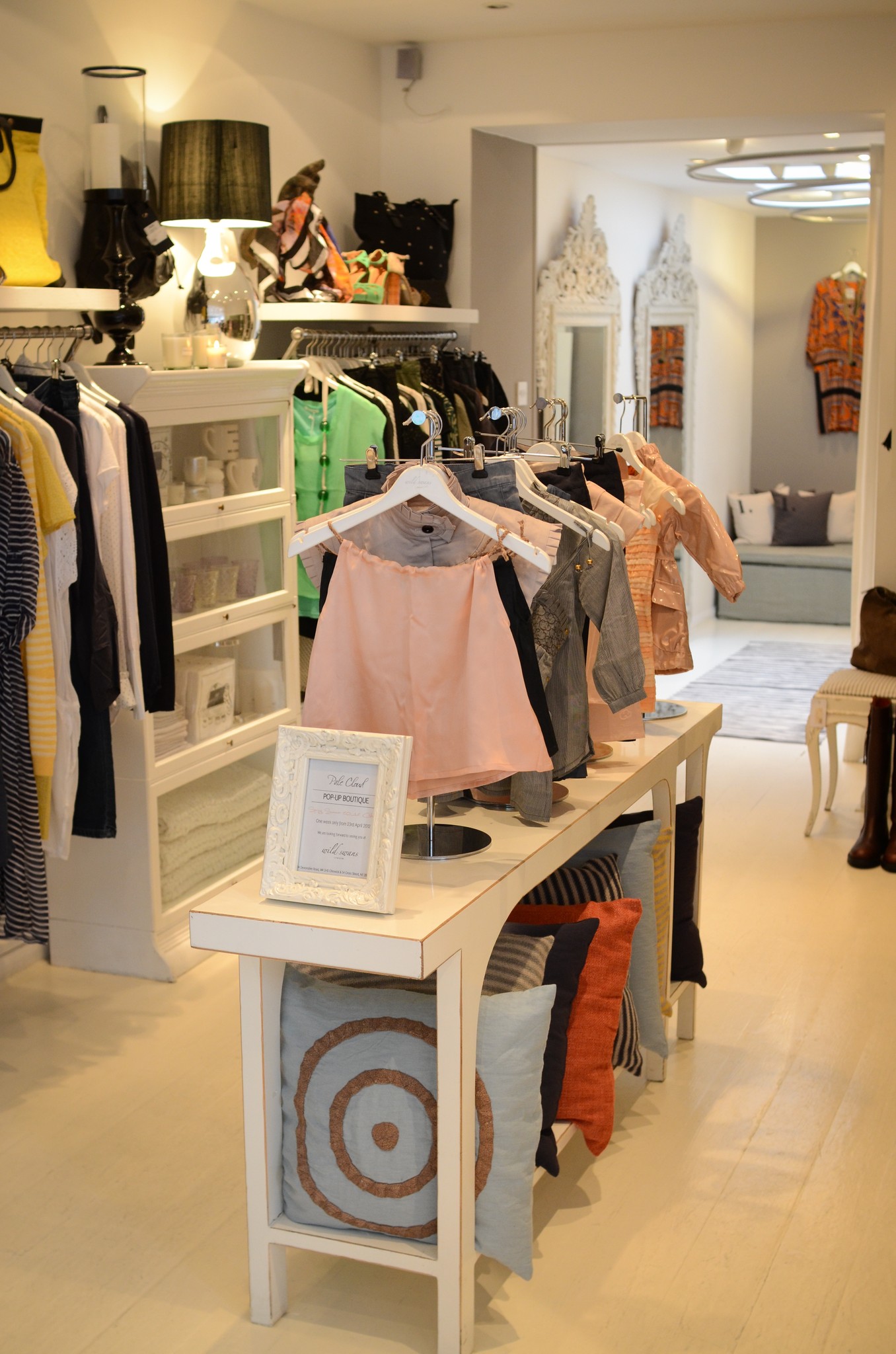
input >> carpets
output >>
[668,637,850,746]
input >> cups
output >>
[168,555,260,615]
[163,332,192,371]
[193,328,221,371]
[150,424,262,507]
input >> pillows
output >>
[292,926,555,1001]
[648,822,680,1015]
[727,481,856,543]
[509,846,642,1082]
[272,956,562,1288]
[545,814,674,1061]
[502,897,646,1158]
[600,794,711,984]
[494,913,597,1181]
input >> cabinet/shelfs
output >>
[42,362,313,983]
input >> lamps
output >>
[153,116,282,361]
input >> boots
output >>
[847,695,894,870]
[881,715,896,873]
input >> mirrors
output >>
[633,213,698,485]
[535,189,624,460]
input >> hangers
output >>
[0,319,124,424]
[287,326,498,407]
[284,388,690,575]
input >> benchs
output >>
[714,541,853,628]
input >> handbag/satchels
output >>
[850,585,895,679]
[354,190,458,281]
[0,113,68,288]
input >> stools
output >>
[801,668,896,837]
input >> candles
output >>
[208,340,228,366]
[183,324,214,367]
[162,331,192,368]
[89,106,124,187]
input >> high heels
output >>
[341,249,391,303]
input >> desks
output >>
[188,689,727,1354]
